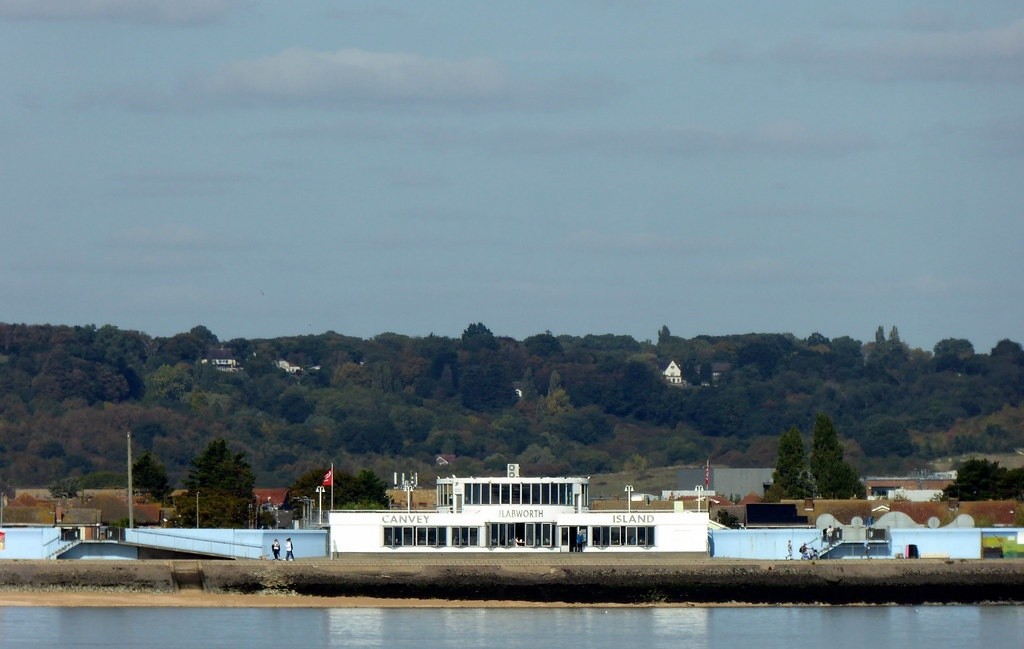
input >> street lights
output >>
[316,485,325,524]
[695,485,704,515]
[624,485,634,515]
[197,491,200,529]
[404,485,414,515]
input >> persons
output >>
[631,536,635,545]
[285,538,294,562]
[273,539,280,561]
[861,538,870,560]
[826,525,834,548]
[786,540,793,560]
[801,543,809,561]
[576,531,584,553]
[452,536,550,547]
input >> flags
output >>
[321,469,331,486]
[705,467,710,484]
